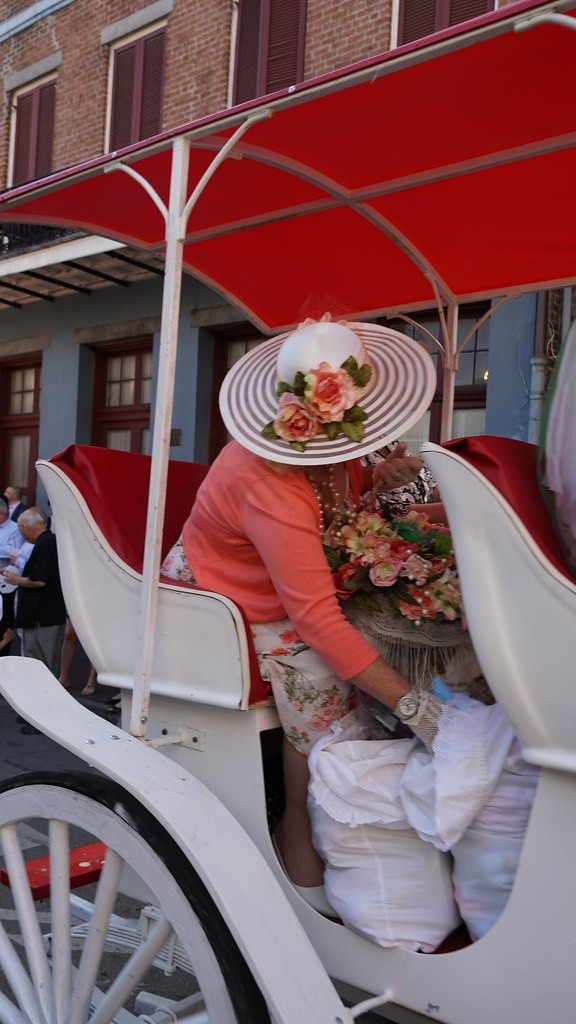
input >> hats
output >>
[218,312,438,466]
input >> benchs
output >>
[36,433,545,711]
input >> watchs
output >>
[393,685,421,721]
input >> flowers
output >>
[261,362,379,452]
[324,499,466,628]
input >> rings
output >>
[393,472,402,481]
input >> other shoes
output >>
[22,724,42,735]
[82,679,97,695]
[16,716,27,723]
[59,680,71,689]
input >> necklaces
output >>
[302,461,358,537]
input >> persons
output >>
[161,439,447,919]
[0,486,33,656]
[0,506,66,736]
[59,610,98,695]
[360,438,447,527]
[46,500,54,534]
[105,692,121,714]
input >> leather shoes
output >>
[271,834,338,917]
[109,704,120,713]
[105,697,121,705]
